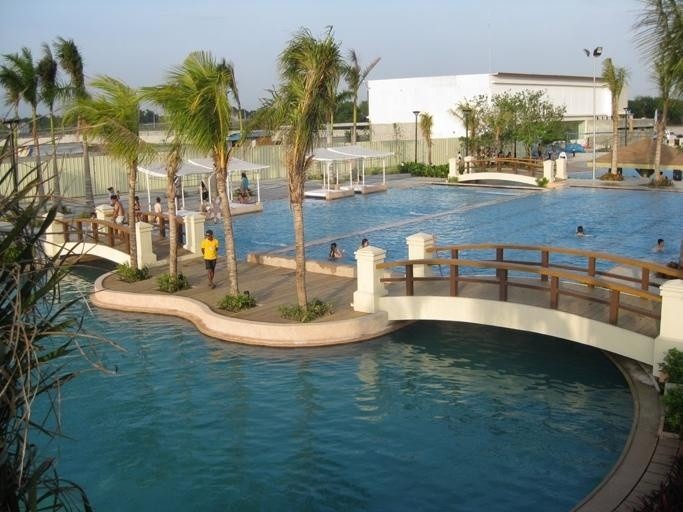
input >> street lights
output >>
[579,44,605,179]
[461,106,471,174]
[412,110,420,163]
[623,108,631,147]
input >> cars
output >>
[531,140,585,158]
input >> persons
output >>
[656,239,664,251]
[458,143,541,159]
[577,226,583,235]
[329,243,342,258]
[201,230,219,288]
[359,238,368,249]
[108,173,252,225]
